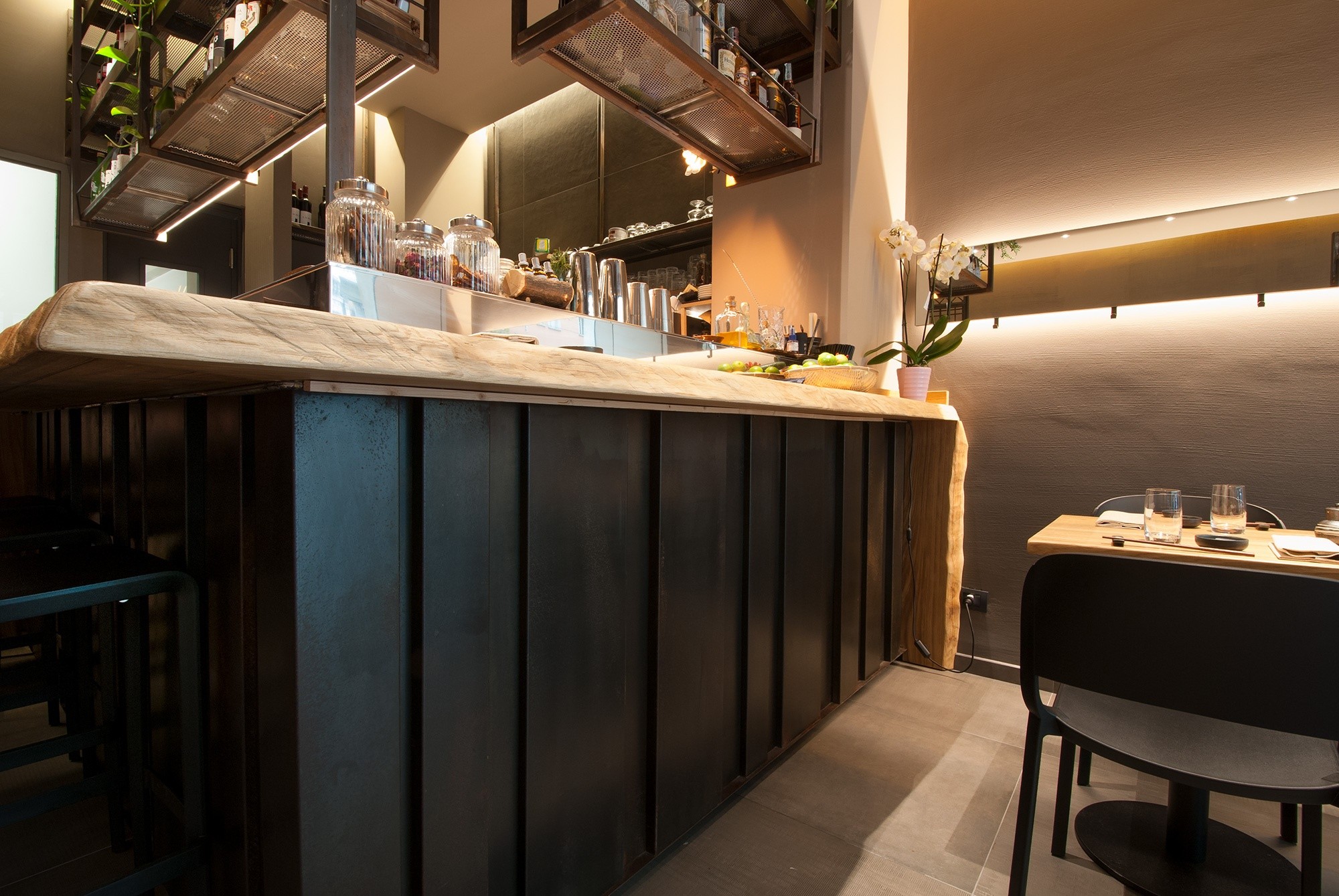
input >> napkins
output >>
[1144,507,1153,519]
[1268,534,1339,565]
[1095,510,1145,530]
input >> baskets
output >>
[737,373,785,380]
[783,365,878,392]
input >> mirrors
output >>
[914,187,1339,326]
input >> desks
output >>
[1027,514,1339,895]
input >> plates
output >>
[697,284,712,300]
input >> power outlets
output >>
[960,586,987,613]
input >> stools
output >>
[0,492,212,896]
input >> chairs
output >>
[1015,494,1339,895]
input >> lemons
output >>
[717,361,781,374]
[786,351,854,370]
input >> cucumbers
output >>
[758,361,786,370]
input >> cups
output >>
[1211,483,1247,534]
[608,222,675,243]
[628,254,700,290]
[795,332,808,355]
[757,304,786,351]
[1144,488,1183,545]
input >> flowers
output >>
[864,220,972,367]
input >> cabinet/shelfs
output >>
[510,0,845,187]
[515,216,711,308]
[929,244,994,322]
[65,0,441,244]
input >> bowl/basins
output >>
[784,365,878,393]
[1195,534,1249,551]
[1183,515,1203,528]
[806,337,822,348]
[693,335,724,343]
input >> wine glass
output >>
[687,195,714,223]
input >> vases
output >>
[896,367,932,403]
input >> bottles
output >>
[714,295,755,349]
[567,250,674,335]
[202,0,269,82]
[695,253,711,286]
[500,258,515,299]
[394,218,450,286]
[153,68,176,135]
[90,114,138,202]
[291,181,312,226]
[318,185,327,229]
[326,176,397,273]
[787,335,798,354]
[443,214,500,296]
[633,0,802,140]
[514,253,560,282]
